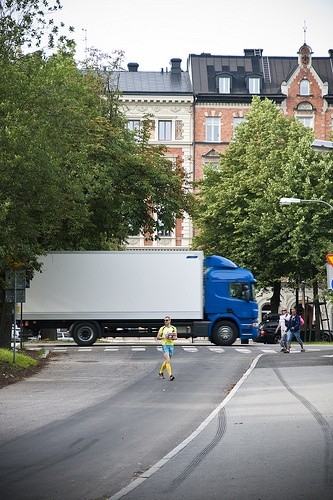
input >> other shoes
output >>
[158,373,165,380]
[284,349,290,353]
[301,349,305,352]
[168,375,175,381]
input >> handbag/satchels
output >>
[284,313,289,327]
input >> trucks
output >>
[11,247,262,347]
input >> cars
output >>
[252,320,282,344]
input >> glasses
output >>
[163,320,169,322]
[282,310,286,312]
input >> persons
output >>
[284,306,305,353]
[156,316,177,381]
[274,309,291,352]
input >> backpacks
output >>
[296,315,305,329]
